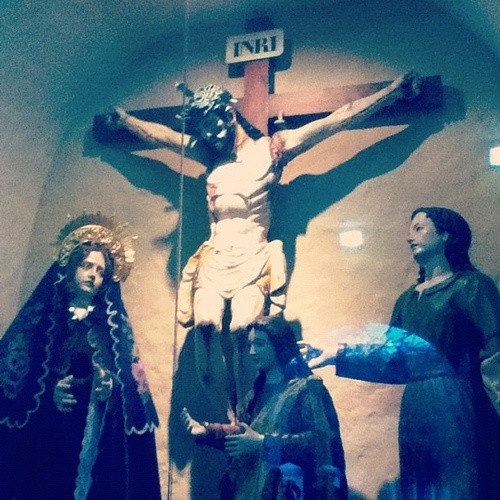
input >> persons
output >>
[0,210,162,499]
[294,205,500,500]
[177,313,349,500]
[104,66,425,425]
